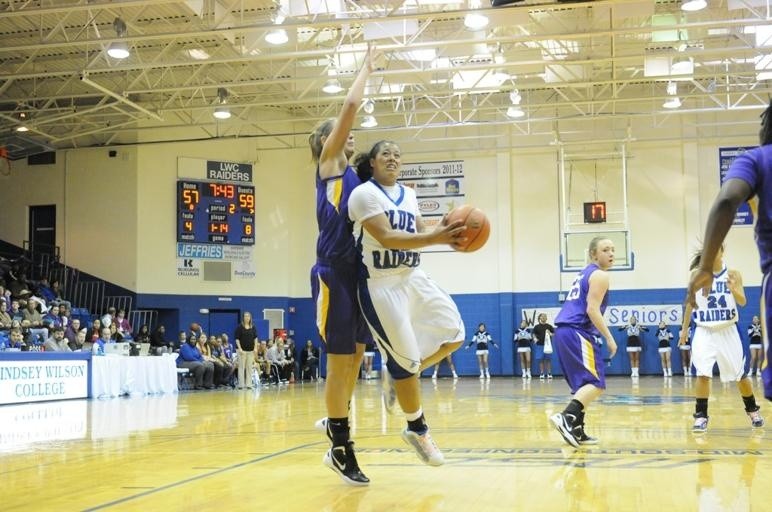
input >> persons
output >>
[0,263,296,390]
[678,241,763,432]
[551,237,618,449]
[688,94,772,402]
[308,39,381,488]
[514,313,532,378]
[432,353,458,379]
[748,315,763,376]
[300,340,320,381]
[528,313,556,379]
[618,315,650,378]
[655,322,675,376]
[464,323,499,378]
[363,342,375,379]
[347,140,465,466]
[680,320,694,376]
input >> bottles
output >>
[1,342,5,352]
[98,346,102,356]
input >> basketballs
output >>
[444,205,489,253]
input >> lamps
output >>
[107,6,131,60]
[663,81,682,109]
[213,88,232,120]
[506,80,525,120]
[361,97,378,128]
[322,55,344,94]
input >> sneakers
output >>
[381,364,399,415]
[756,371,761,376]
[195,377,320,392]
[629,370,694,377]
[314,417,356,452]
[572,423,599,444]
[429,368,557,380]
[367,375,370,379]
[401,425,445,467]
[323,442,370,487]
[747,370,753,376]
[549,410,581,448]
[745,409,763,429]
[691,414,710,434]
[365,374,368,379]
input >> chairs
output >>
[71,308,98,342]
[162,352,190,390]
